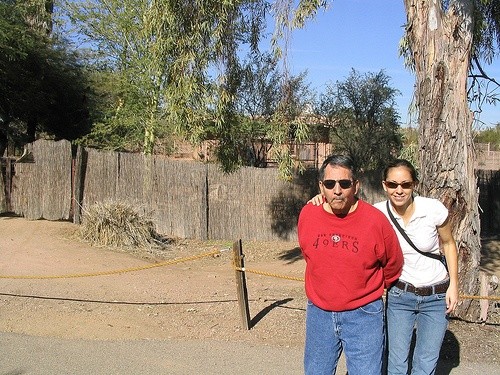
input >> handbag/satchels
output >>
[440,255,448,269]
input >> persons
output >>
[305,158,462,375]
[296,153,404,375]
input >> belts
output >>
[394,279,450,296]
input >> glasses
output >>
[321,178,354,190]
[384,181,414,190]
